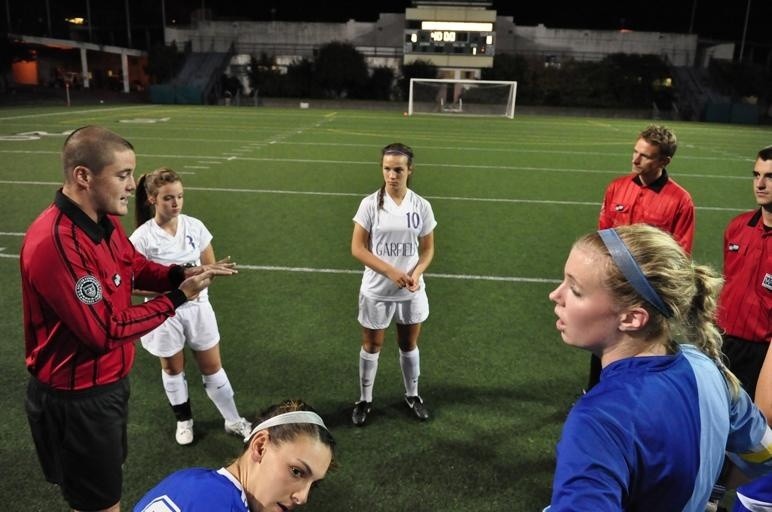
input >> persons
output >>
[350,144,436,427]
[19,125,238,512]
[577,125,695,405]
[128,167,252,448]
[130,398,337,512]
[541,223,772,512]
[716,334,772,512]
[690,145,772,512]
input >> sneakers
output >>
[352,399,373,426]
[225,416,252,438]
[175,418,194,446]
[403,393,429,420]
